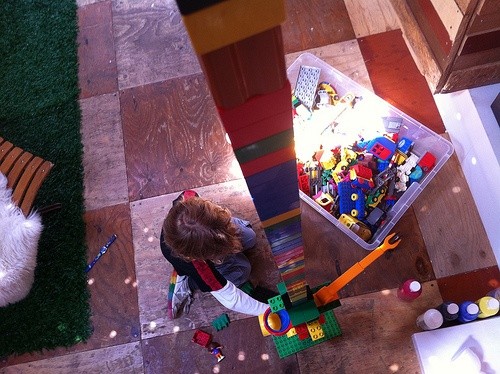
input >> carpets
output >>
[0,0,94,362]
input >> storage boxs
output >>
[225,51,455,250]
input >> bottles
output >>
[416,308,443,331]
[459,301,479,324]
[397,280,422,303]
[474,296,500,319]
[437,300,459,324]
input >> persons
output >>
[159,190,271,322]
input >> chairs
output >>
[0,136,54,308]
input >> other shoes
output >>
[167,270,193,320]
